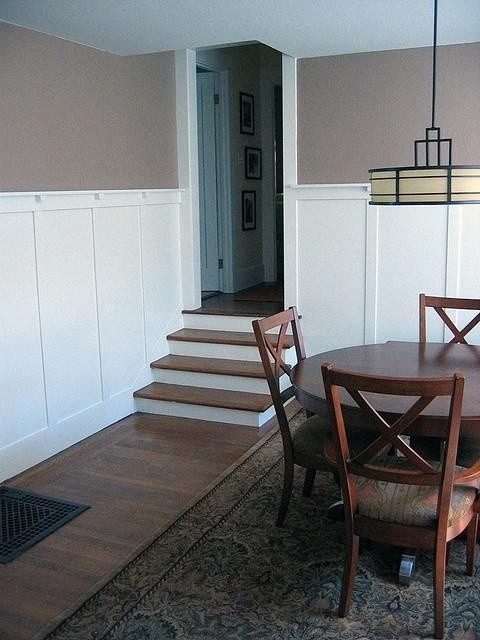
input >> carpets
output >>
[31,400,480,640]
[0,483,90,563]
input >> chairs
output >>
[255,305,395,529]
[321,363,479,639]
[419,293,480,343]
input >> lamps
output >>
[368,0,480,205]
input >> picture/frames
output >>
[239,91,263,231]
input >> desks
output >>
[290,342,478,465]
